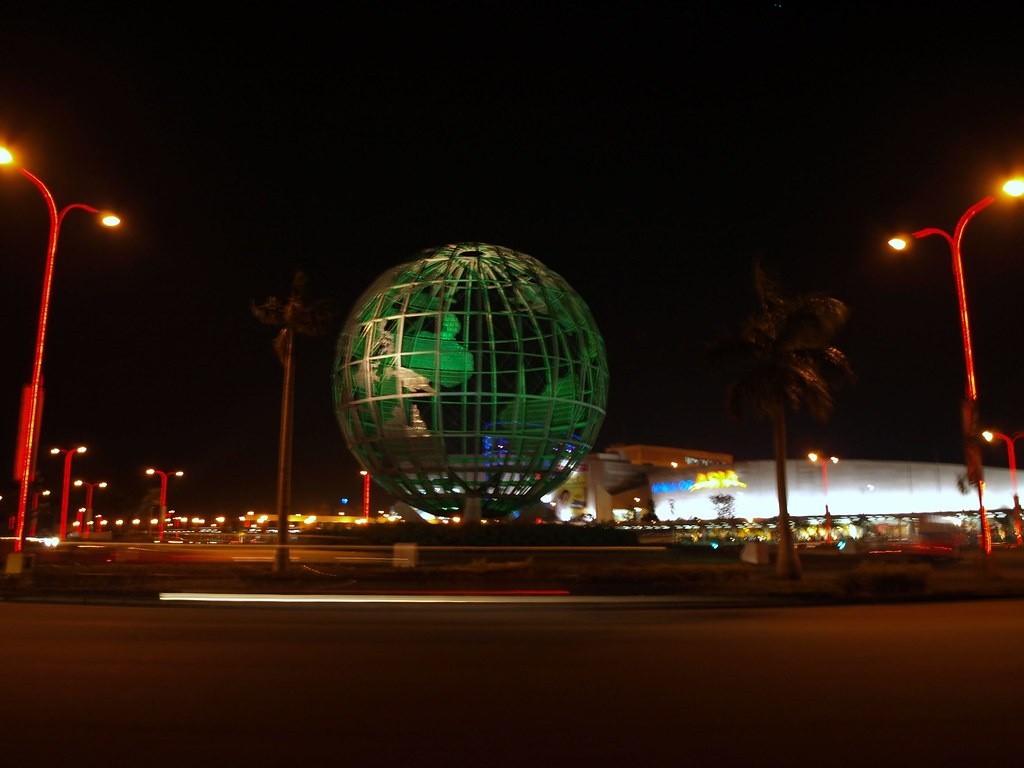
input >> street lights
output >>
[146,468,183,541]
[49,446,88,542]
[982,429,1023,547]
[808,452,840,519]
[888,173,1024,555]
[74,481,108,541]
[28,490,51,538]
[0,146,121,553]
